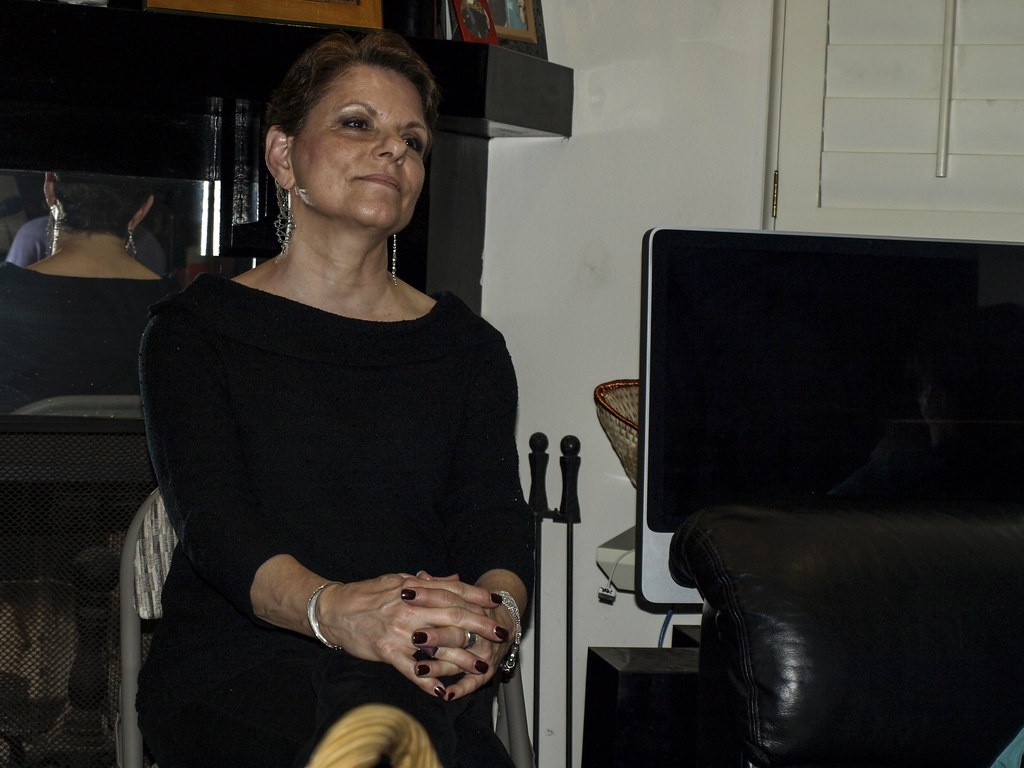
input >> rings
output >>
[463,630,476,649]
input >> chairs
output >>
[117,484,535,766]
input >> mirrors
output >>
[1,168,223,413]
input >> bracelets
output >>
[306,581,344,650]
[494,590,522,672]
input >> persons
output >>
[134,25,537,768]
[0,170,176,413]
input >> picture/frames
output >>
[452,0,499,44]
[487,0,537,44]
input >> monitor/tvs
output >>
[636,228,1024,612]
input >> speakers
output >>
[0,414,162,768]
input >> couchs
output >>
[668,501,1023,768]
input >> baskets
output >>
[595,378,637,491]
